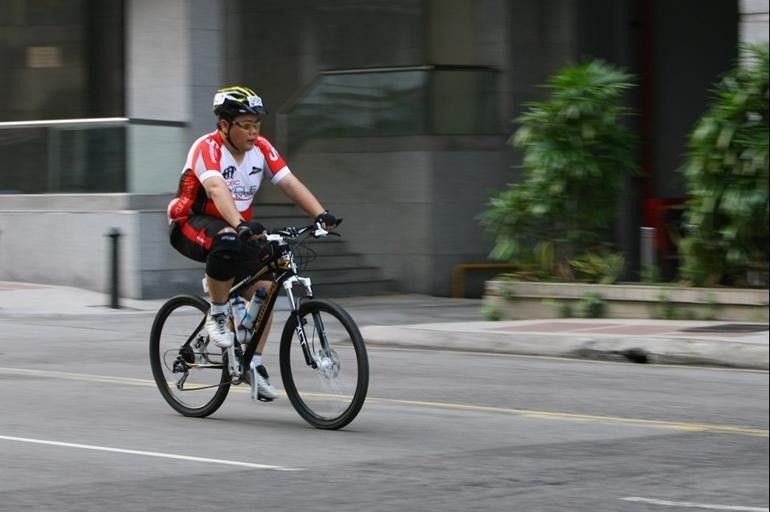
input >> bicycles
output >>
[150,214,368,430]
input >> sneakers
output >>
[204,308,233,348]
[246,364,277,399]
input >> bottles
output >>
[231,294,252,344]
[239,286,267,330]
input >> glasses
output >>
[232,120,261,130]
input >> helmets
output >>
[213,86,266,116]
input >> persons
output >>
[167,85,337,401]
